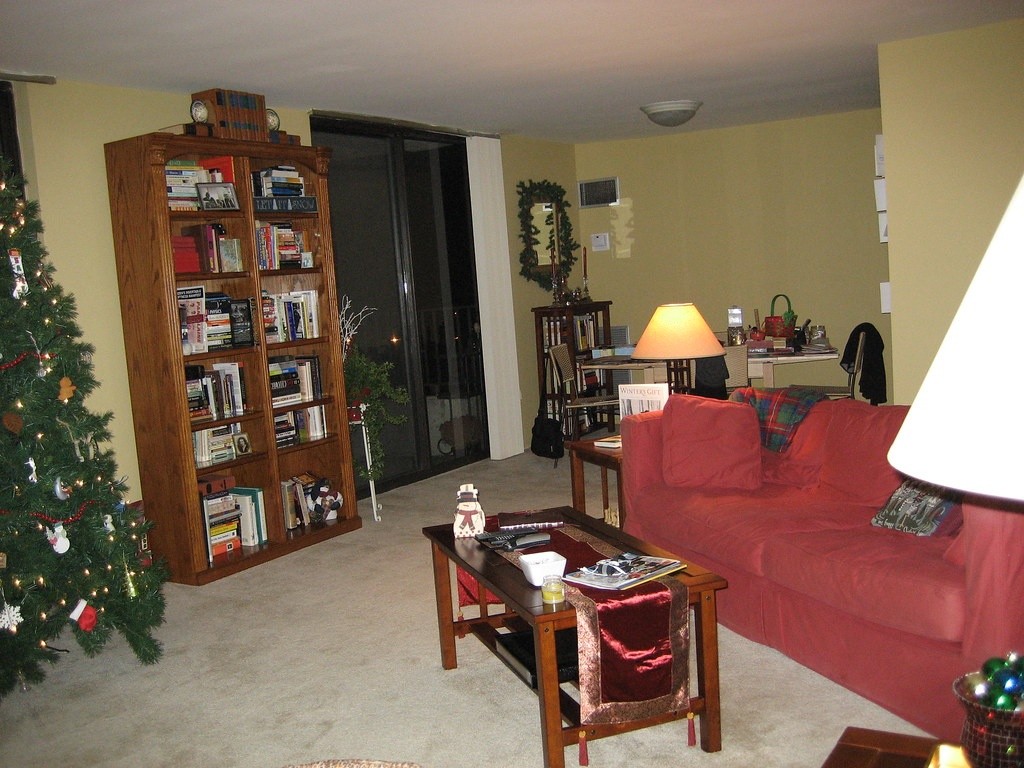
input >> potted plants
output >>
[440,372,480,446]
[338,292,410,481]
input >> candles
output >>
[551,248,556,278]
[583,247,587,277]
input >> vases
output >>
[953,669,1024,768]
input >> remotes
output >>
[475,528,552,552]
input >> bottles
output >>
[541,575,564,603]
[809,326,826,342]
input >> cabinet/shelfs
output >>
[102,131,362,587]
[437,394,482,456]
[879,19,1024,408]
[531,301,616,440]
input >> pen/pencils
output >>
[749,325,754,334]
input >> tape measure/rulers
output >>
[754,309,760,330]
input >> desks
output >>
[579,348,839,388]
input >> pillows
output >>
[870,476,964,537]
[662,395,763,490]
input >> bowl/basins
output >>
[519,551,567,586]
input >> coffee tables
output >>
[422,506,727,768]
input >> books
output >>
[739,338,775,353]
[158,148,255,470]
[560,550,687,592]
[204,484,270,563]
[280,469,317,529]
[543,315,601,434]
[246,159,329,450]
[496,509,565,531]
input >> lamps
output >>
[888,175,1024,502]
[639,100,703,128]
[630,303,727,395]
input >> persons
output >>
[238,438,249,453]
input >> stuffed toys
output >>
[57,377,77,399]
[104,515,116,534]
[44,521,70,554]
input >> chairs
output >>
[549,344,619,468]
[683,346,750,389]
[790,322,888,406]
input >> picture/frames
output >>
[232,432,253,457]
[194,182,240,212]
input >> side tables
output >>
[564,434,626,532]
[823,726,962,768]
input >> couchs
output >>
[620,386,1023,743]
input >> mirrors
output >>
[516,179,582,292]
